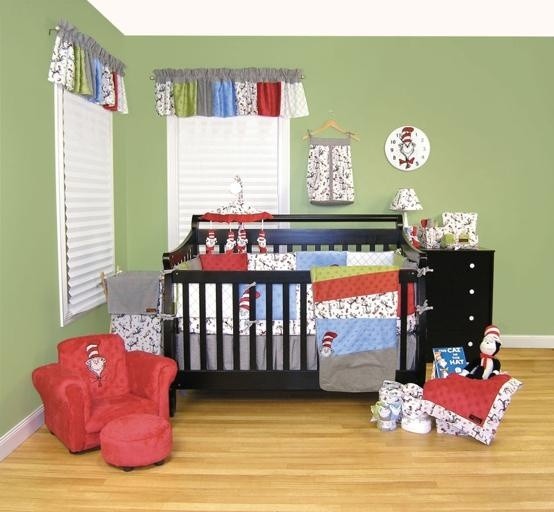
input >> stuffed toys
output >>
[458,326,502,381]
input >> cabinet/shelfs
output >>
[418,246,500,363]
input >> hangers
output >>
[302,110,361,144]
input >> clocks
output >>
[384,124,432,172]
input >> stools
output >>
[99,411,174,472]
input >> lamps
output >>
[391,187,423,236]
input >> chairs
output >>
[31,333,178,454]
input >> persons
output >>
[257,230,267,253]
[223,231,237,254]
[235,227,247,254]
[204,229,218,255]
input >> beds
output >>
[161,214,429,419]
